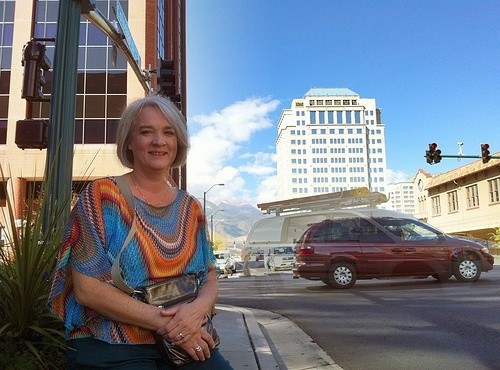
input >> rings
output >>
[179,333,185,338]
[194,346,201,352]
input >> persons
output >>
[222,254,234,279]
[46,97,235,370]
[400,229,407,240]
[266,256,296,271]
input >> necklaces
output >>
[129,173,170,207]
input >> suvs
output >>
[268,245,295,272]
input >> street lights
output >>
[203,183,224,219]
[211,209,225,254]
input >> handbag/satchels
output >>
[142,275,220,367]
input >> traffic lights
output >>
[426,142,442,164]
[22,42,51,101]
[481,143,491,164]
[160,57,176,97]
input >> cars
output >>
[214,249,230,272]
[294,216,494,288]
